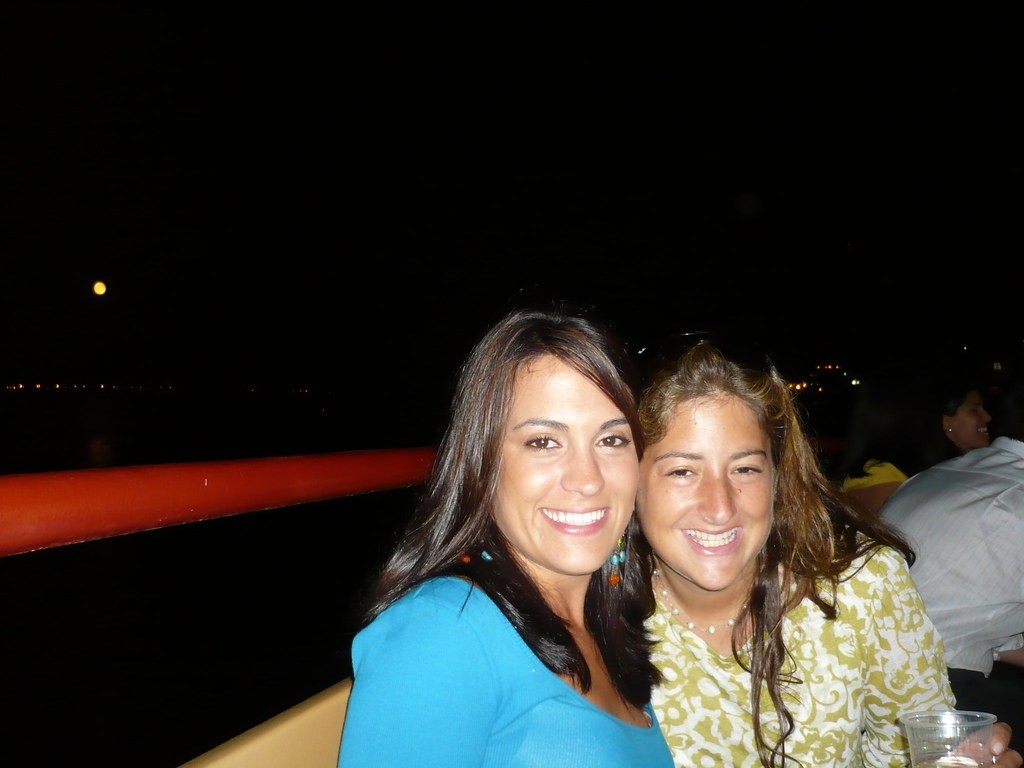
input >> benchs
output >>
[175,675,355,768]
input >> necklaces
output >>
[654,568,747,633]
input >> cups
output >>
[899,710,998,768]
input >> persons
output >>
[333,313,675,768]
[606,335,1024,768]
[841,370,1024,756]
